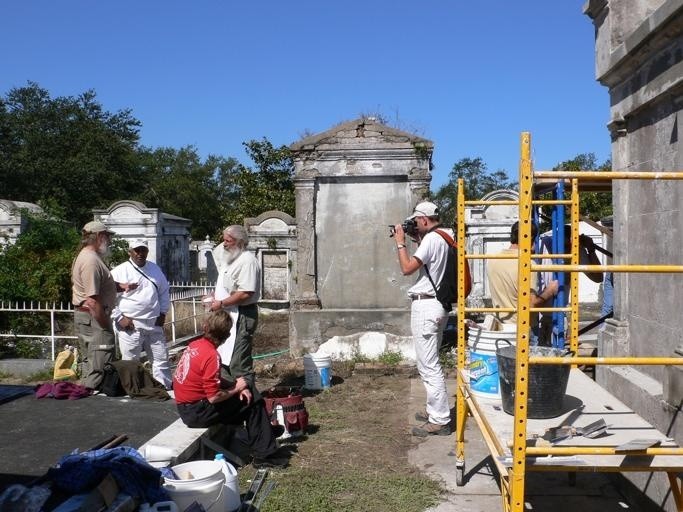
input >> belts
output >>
[76,306,91,311]
[411,295,434,300]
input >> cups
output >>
[204,298,213,313]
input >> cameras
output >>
[388,221,418,237]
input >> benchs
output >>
[51,417,243,512]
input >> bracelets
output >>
[220,300,222,309]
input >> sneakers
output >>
[412,421,451,436]
[252,457,289,470]
[415,408,429,421]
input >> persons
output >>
[172,311,289,468]
[391,200,456,435]
[485,220,613,347]
[71,221,139,389]
[110,239,175,398]
[209,224,261,384]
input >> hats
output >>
[130,239,148,250]
[406,202,440,220]
[82,222,116,235]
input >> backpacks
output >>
[423,230,471,304]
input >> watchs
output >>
[396,245,406,249]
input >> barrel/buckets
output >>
[468,321,531,399]
[213,454,241,512]
[261,389,304,433]
[163,460,224,512]
[303,354,332,390]
[495,337,574,418]
